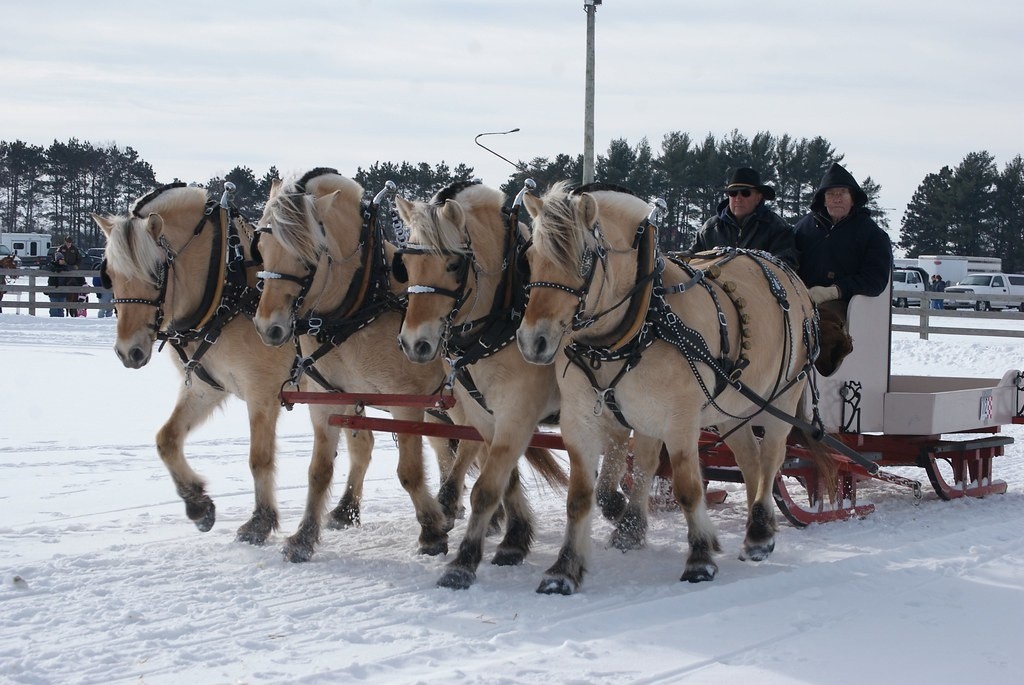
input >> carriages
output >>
[90,167,1023,596]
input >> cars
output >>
[0,244,22,267]
[88,248,105,257]
[47,247,102,271]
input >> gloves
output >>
[808,285,839,304]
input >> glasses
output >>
[728,189,757,198]
[825,191,850,200]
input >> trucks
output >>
[892,270,950,308]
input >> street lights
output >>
[583,0,602,184]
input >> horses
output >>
[89,172,849,601]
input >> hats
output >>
[65,236,73,242]
[714,167,776,200]
[55,252,64,261]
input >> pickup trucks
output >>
[943,274,1024,312]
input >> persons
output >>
[44,251,70,317]
[794,161,894,377]
[928,274,945,309]
[666,165,800,274]
[57,235,86,285]
[98,259,114,318]
